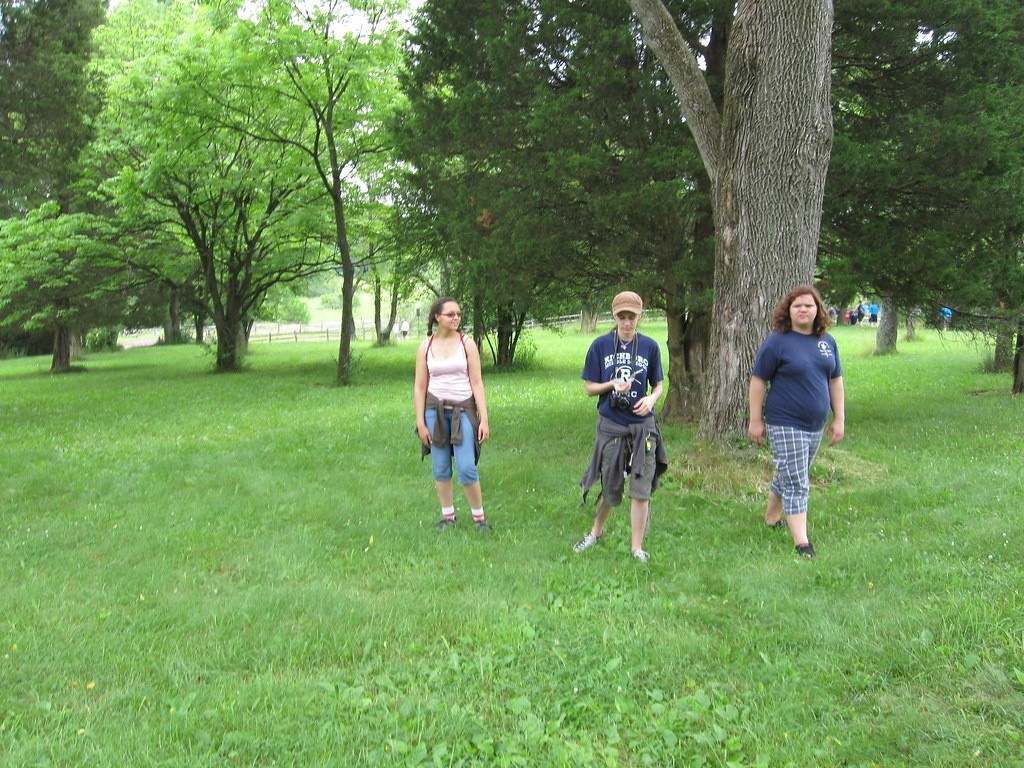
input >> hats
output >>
[611,291,643,316]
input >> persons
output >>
[828,296,879,326]
[574,290,664,564]
[940,305,952,332]
[413,297,492,537]
[747,287,845,559]
[392,319,409,341]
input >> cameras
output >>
[611,396,630,409]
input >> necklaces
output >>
[617,336,634,350]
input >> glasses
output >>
[439,311,462,318]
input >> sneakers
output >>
[573,533,600,553]
[632,548,650,562]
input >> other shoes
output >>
[794,541,815,557]
[436,517,458,530]
[474,519,487,530]
[769,519,784,531]
